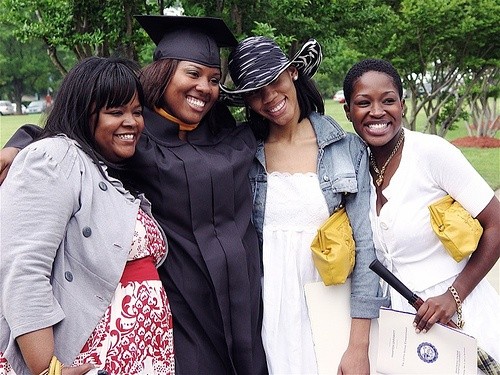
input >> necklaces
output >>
[371,128,404,187]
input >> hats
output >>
[134,15,239,70]
[219,35,322,93]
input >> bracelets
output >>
[33,364,65,375]
[448,285,466,330]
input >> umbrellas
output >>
[369,258,500,375]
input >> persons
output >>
[0,15,269,375]
[343,60,500,375]
[220,35,388,375]
[0,58,176,375]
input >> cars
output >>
[333,90,345,102]
[0,100,53,115]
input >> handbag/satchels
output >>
[428,195,483,263]
[309,207,355,286]
